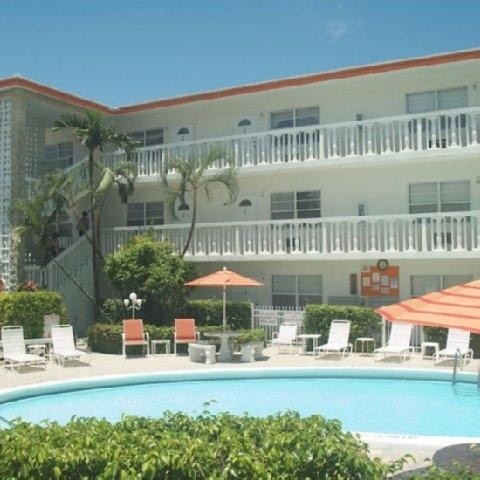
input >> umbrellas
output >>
[183,266,265,331]
[374,278,480,336]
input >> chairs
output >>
[373,321,416,363]
[313,319,353,360]
[433,327,474,370]
[50,323,82,368]
[1,325,49,375]
[172,319,201,356]
[270,321,298,353]
[119,319,150,359]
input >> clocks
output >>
[377,259,388,271]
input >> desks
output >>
[298,334,323,356]
[353,337,376,356]
[203,331,244,361]
[420,342,440,362]
[26,345,47,357]
[151,340,170,356]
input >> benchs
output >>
[186,341,216,364]
[238,341,264,362]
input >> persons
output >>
[77,211,90,237]
